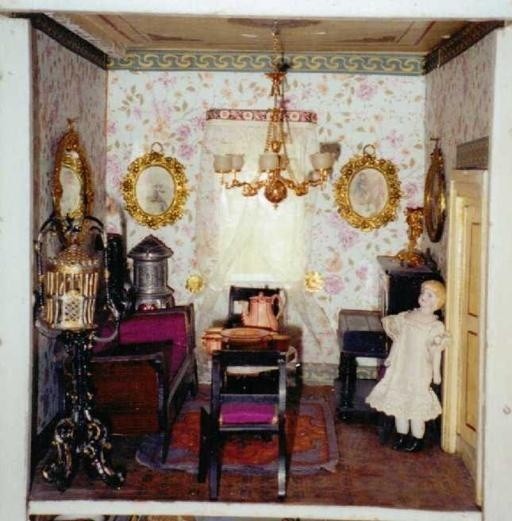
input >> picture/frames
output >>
[333,145,402,232]
[120,142,189,229]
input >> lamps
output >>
[213,19,340,209]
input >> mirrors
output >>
[422,137,446,243]
[53,118,95,247]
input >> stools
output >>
[337,309,393,424]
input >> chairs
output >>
[197,285,290,501]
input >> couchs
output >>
[89,303,199,465]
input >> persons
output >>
[364,278,449,453]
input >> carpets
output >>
[135,393,340,478]
